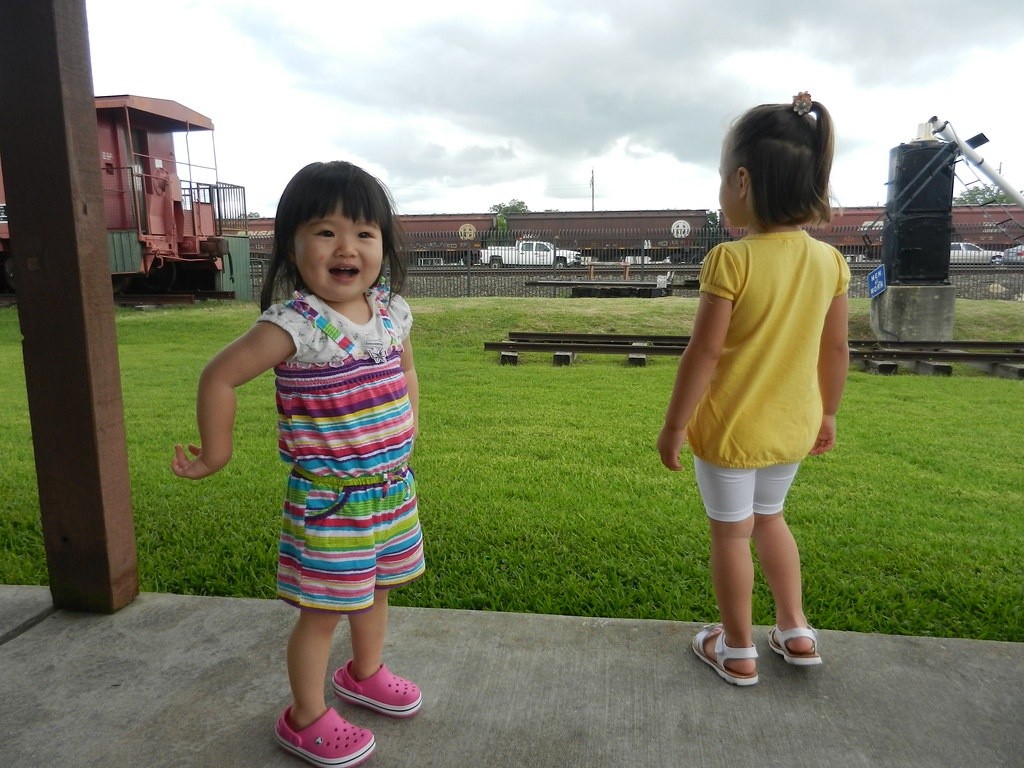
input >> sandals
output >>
[696,625,759,685]
[770,619,824,669]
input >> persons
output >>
[173,160,433,768]
[654,89,848,688]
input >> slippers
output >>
[276,706,374,766]
[331,660,421,716]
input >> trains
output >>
[1,94,232,304]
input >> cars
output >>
[951,241,1006,267]
[1002,244,1024,264]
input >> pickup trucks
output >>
[479,235,582,269]
